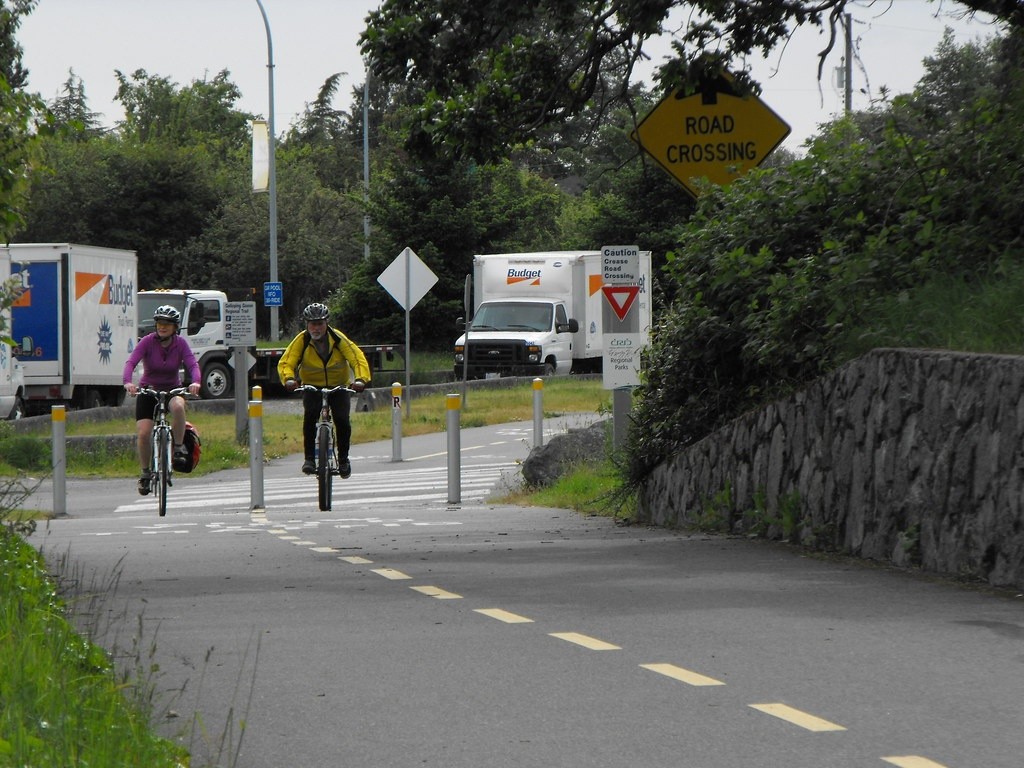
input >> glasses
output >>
[156,319,175,326]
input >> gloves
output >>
[286,380,299,392]
[351,381,367,393]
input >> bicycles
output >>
[129,386,199,515]
[293,383,357,512]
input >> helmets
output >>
[154,304,181,323]
[303,303,330,321]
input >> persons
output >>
[277,302,371,479]
[122,304,201,495]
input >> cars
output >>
[0,343,27,417]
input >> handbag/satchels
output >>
[172,422,201,474]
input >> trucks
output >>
[133,290,407,398]
[0,243,140,406]
[453,250,653,378]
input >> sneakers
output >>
[173,445,186,463]
[302,459,316,472]
[338,457,352,479]
[137,470,152,495]
